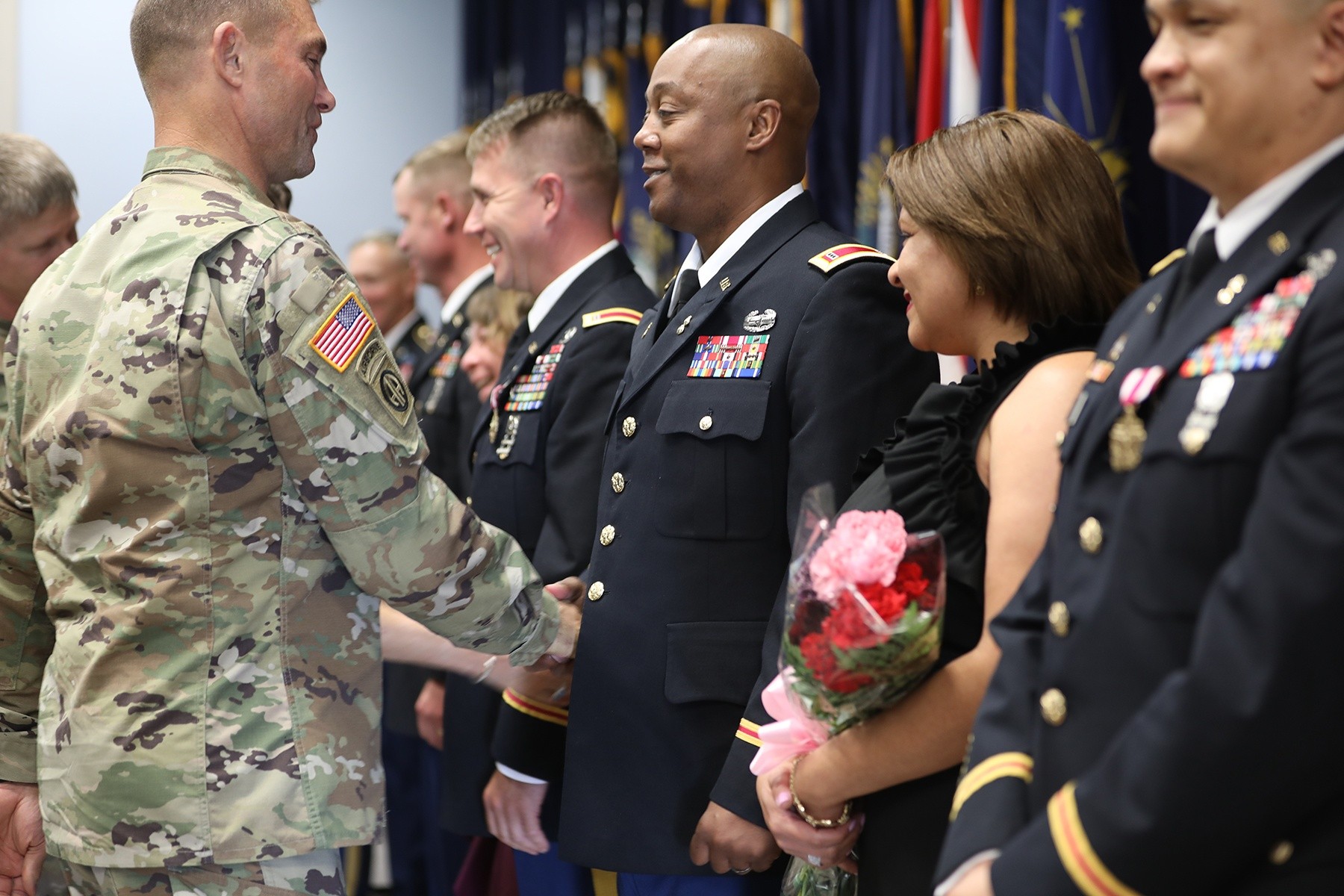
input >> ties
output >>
[664,270,699,326]
[1165,228,1218,327]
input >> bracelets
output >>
[788,756,852,829]
[474,656,495,685]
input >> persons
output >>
[346,231,438,393]
[413,92,662,895]
[929,1,1344,896]
[0,0,583,896]
[460,281,533,405]
[0,0,1344,896]
[481,21,940,895]
[0,131,81,357]
[754,108,1132,895]
[381,120,498,742]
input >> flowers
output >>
[749,481,949,896]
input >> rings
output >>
[729,867,753,875]
[807,855,824,866]
